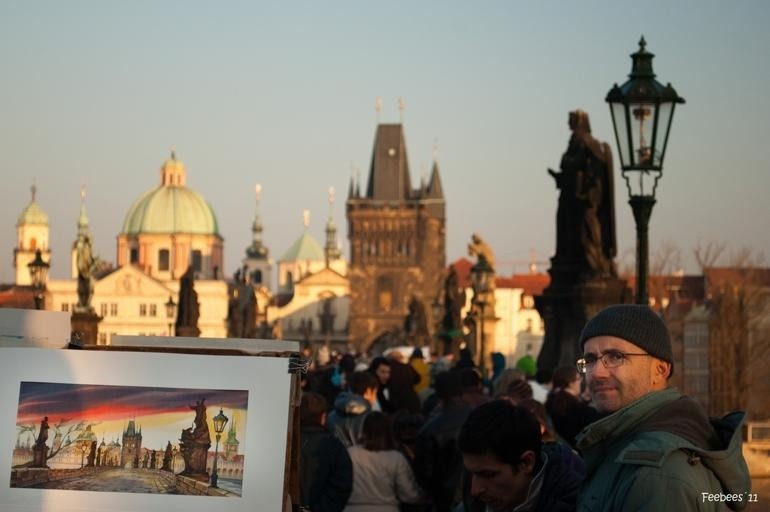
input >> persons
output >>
[295,304,752,511]
[548,107,613,280]
[466,231,495,281]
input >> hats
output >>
[579,304,672,363]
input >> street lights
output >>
[158,444,164,468]
[164,293,180,336]
[81,442,88,467]
[208,403,230,484]
[26,246,51,311]
[172,446,179,473]
[467,253,501,368]
[101,439,105,460]
[604,37,689,306]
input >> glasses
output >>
[575,351,649,374]
